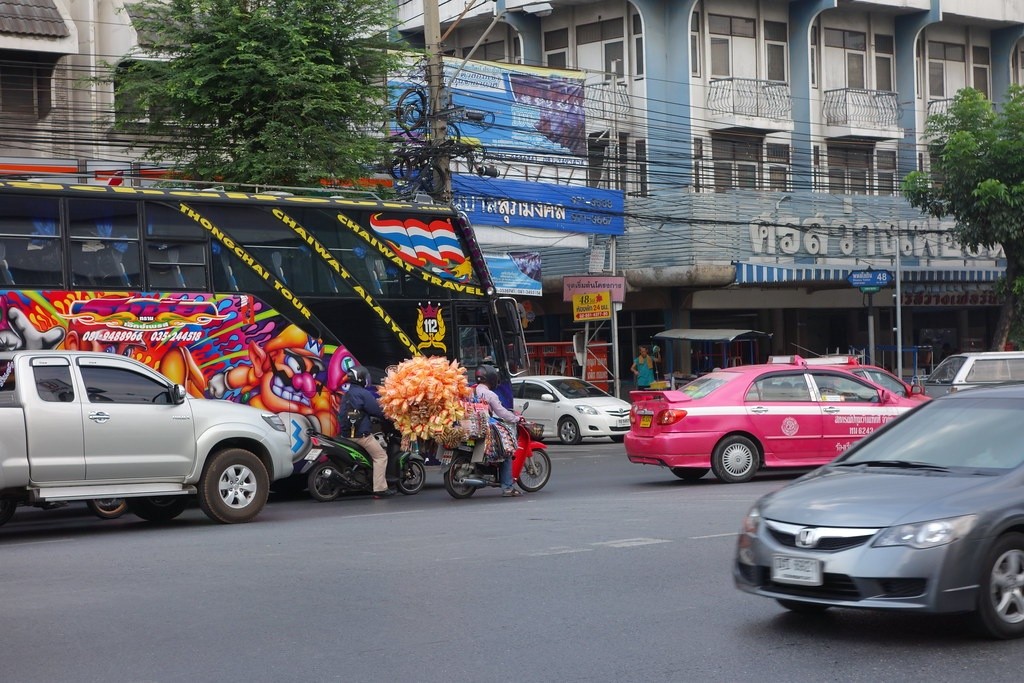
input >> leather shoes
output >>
[373,488,397,498]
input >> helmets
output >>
[347,366,371,389]
[475,364,498,391]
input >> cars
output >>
[732,380,1024,641]
[624,355,923,484]
[924,352,1024,397]
[510,375,633,445]
[803,355,933,404]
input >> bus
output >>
[0,171,529,415]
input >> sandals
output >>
[502,487,524,497]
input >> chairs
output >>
[0,237,387,299]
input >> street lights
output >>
[428,3,553,202]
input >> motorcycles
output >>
[300,417,426,501]
[440,403,551,499]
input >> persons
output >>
[920,338,943,375]
[338,367,399,499]
[629,346,661,391]
[466,364,526,497]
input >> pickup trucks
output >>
[0,350,294,527]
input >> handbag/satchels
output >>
[344,391,364,430]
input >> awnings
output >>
[733,261,1007,287]
[483,253,543,297]
